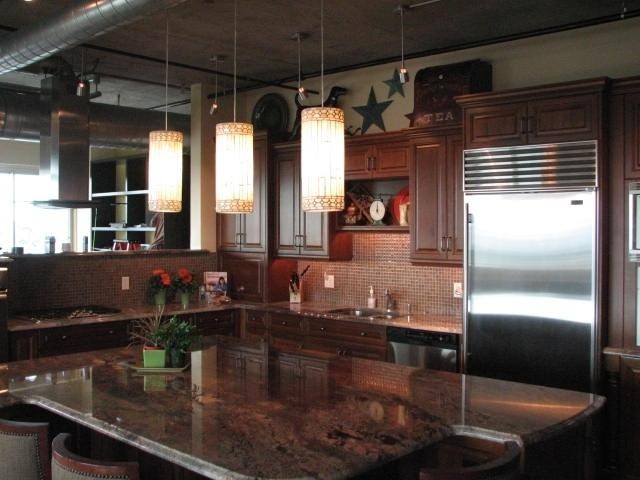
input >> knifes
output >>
[289,264,311,291]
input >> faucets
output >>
[383,287,396,310]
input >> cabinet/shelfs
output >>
[346,133,409,180]
[270,146,354,261]
[8,330,38,361]
[38,321,129,361]
[245,309,269,334]
[270,312,303,338]
[129,314,198,348]
[90,189,158,249]
[409,128,464,267]
[198,308,240,337]
[217,137,270,253]
[303,317,387,364]
[455,90,602,145]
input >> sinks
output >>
[341,308,372,318]
[369,310,399,320]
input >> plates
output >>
[251,94,288,131]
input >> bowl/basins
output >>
[140,244,150,250]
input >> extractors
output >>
[26,75,131,210]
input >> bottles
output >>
[407,303,412,314]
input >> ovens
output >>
[384,326,460,374]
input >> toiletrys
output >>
[367,285,377,308]
[198,285,206,306]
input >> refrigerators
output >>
[460,139,598,393]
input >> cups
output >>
[399,202,408,227]
[62,242,71,252]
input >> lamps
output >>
[290,31,311,99]
[207,55,225,116]
[215,0,254,214]
[396,4,410,84]
[147,7,183,213]
[75,44,89,96]
[299,0,346,212]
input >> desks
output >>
[1,342,616,480]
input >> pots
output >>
[113,239,141,250]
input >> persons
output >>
[214,277,227,292]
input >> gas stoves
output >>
[32,303,124,349]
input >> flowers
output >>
[172,266,202,295]
[146,268,172,293]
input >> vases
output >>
[180,294,191,304]
[154,290,167,306]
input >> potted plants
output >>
[143,312,205,369]
[121,305,168,369]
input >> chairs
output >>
[51,433,141,480]
[416,441,522,480]
[0,418,51,480]
